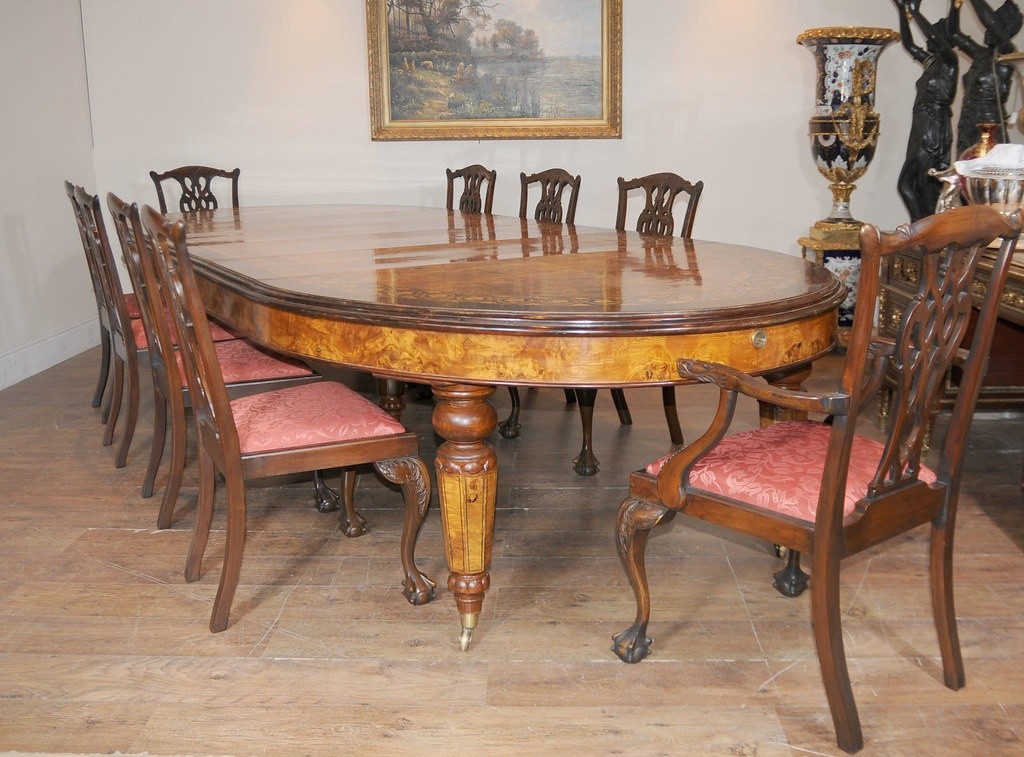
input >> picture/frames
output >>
[366,2,624,141]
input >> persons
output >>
[893,0,1024,223]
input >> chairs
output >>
[105,193,337,529]
[500,169,581,436]
[445,164,500,218]
[150,165,244,221]
[144,207,438,632]
[555,172,705,474]
[76,185,254,446]
[64,181,181,425]
[610,203,1014,753]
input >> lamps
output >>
[952,143,1024,249]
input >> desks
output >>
[119,204,850,650]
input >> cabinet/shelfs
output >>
[869,236,1024,452]
